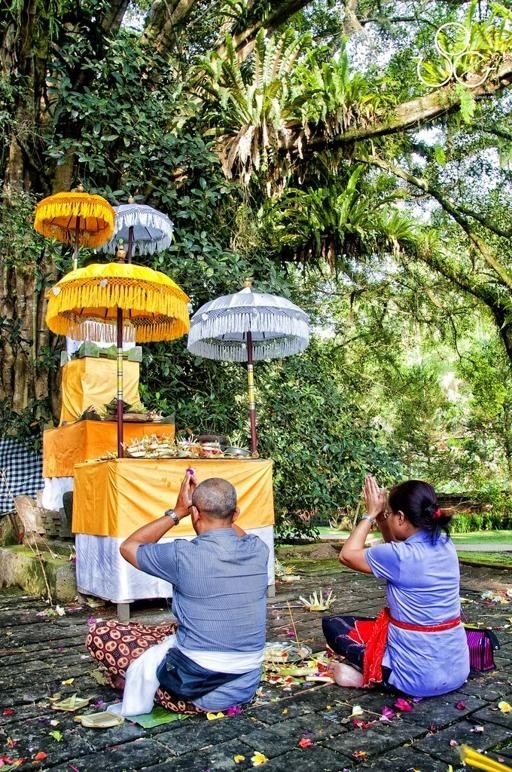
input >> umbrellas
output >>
[187,277,311,451]
[34,184,115,270]
[100,195,173,264]
[43,246,192,458]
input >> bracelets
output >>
[361,515,375,526]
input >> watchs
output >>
[165,509,179,526]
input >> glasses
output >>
[187,504,200,515]
[383,509,394,518]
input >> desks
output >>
[40,418,176,512]
[70,455,276,622]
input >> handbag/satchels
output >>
[464,626,500,673]
[156,651,247,702]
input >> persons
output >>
[85,468,269,715]
[322,474,471,698]
